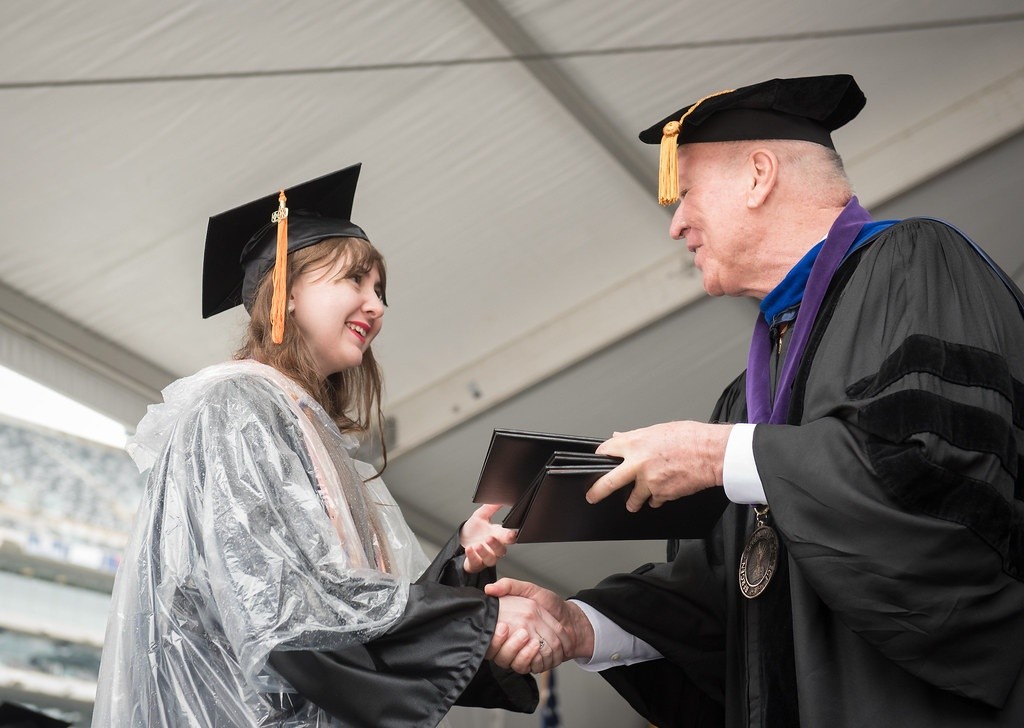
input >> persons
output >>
[482,75,1024,728]
[88,163,572,728]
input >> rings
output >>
[539,639,544,648]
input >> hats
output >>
[198,161,373,346]
[639,72,867,203]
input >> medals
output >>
[739,527,778,598]
